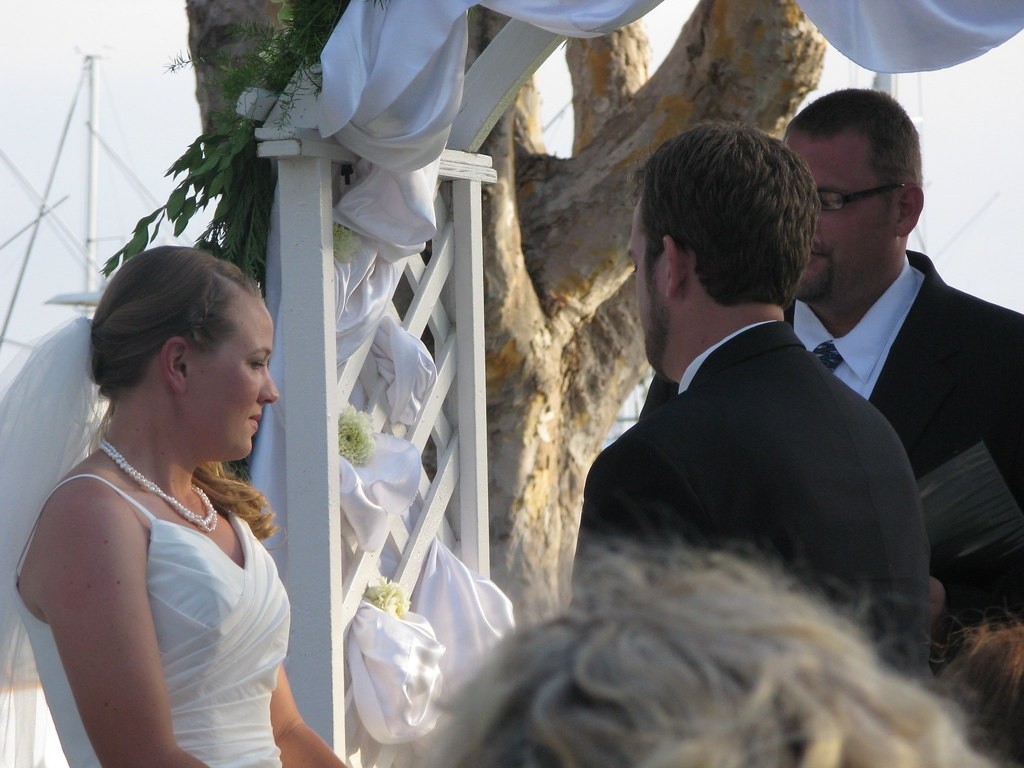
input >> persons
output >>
[574,121,948,706]
[392,535,991,766]
[0,245,351,768]
[642,87,1022,672]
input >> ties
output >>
[814,343,843,373]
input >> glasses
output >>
[817,181,905,211]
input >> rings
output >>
[99,440,220,532]
